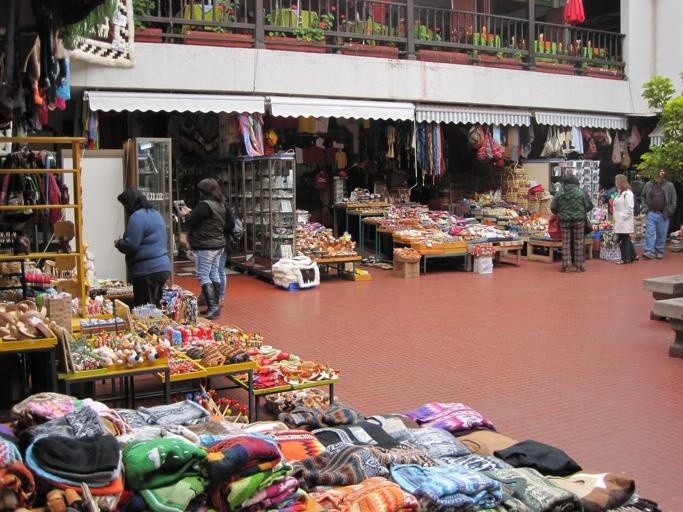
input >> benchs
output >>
[642,274,682,358]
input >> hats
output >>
[24,406,103,442]
[528,181,538,189]
[538,191,553,201]
[24,435,121,496]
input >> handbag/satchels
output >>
[584,217,592,235]
[547,215,561,239]
[473,126,484,149]
[474,128,502,160]
[467,124,481,145]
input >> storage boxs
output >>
[391,247,420,277]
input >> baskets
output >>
[600,247,622,261]
[493,160,530,210]
[527,197,540,213]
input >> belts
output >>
[649,209,662,214]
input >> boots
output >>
[200,283,219,319]
[199,282,220,315]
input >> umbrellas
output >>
[562,1,587,56]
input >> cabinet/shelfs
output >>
[331,198,597,273]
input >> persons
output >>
[550,171,591,271]
[642,169,678,261]
[112,186,171,307]
[632,173,645,216]
[197,248,226,304]
[180,178,235,319]
[579,183,594,270]
[611,173,639,266]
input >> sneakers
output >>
[642,252,653,259]
[560,267,568,272]
[615,260,624,264]
[575,266,585,272]
[655,253,662,258]
